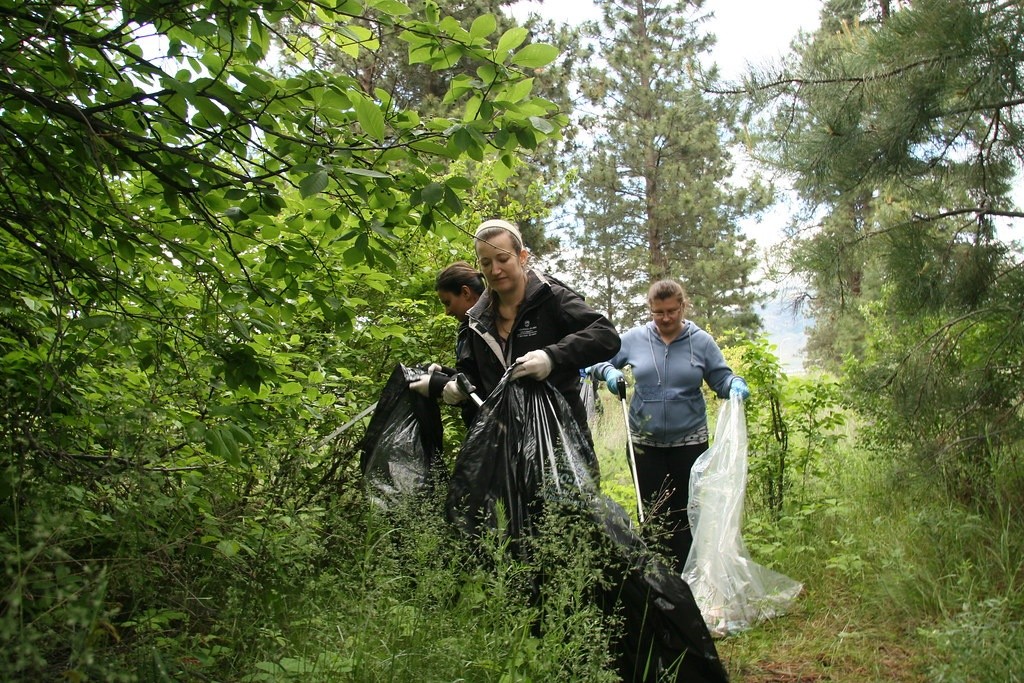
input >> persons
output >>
[437,219,624,464]
[584,280,752,577]
[408,262,490,431]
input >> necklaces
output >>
[499,321,511,333]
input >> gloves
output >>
[409,374,432,398]
[428,364,442,376]
[730,379,750,401]
[602,367,626,394]
[510,349,551,380]
[442,381,476,405]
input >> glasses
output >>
[650,303,683,317]
